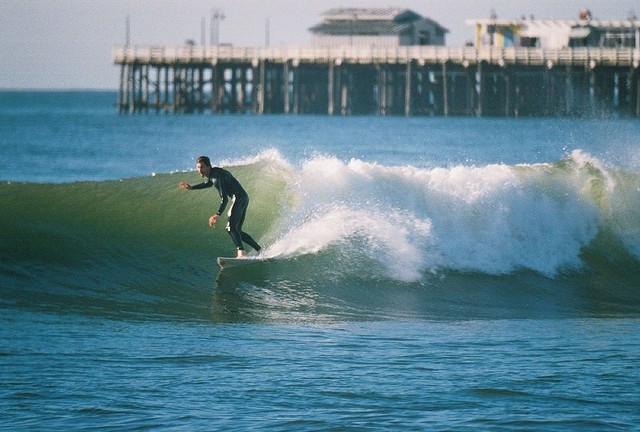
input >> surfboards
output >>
[216,256,269,266]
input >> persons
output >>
[180,156,264,259]
